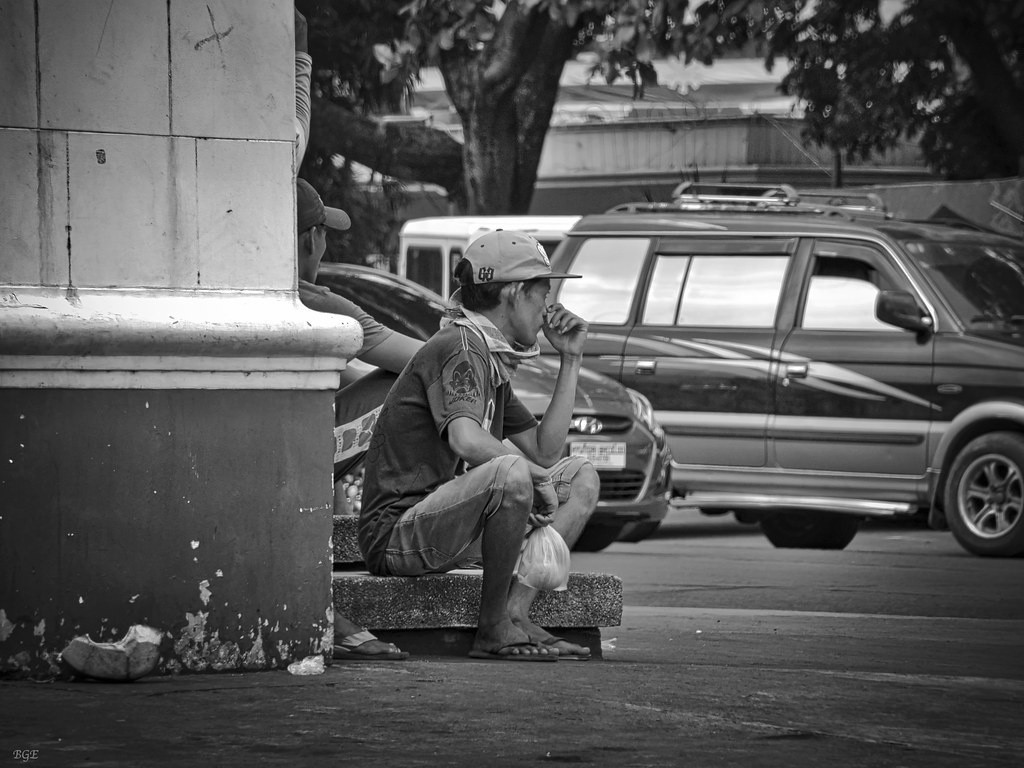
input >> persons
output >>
[334,610,412,660]
[295,173,428,484]
[361,229,602,664]
[294,10,311,169]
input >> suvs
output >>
[526,176,1024,561]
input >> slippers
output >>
[335,626,409,660]
[469,634,559,661]
[540,637,592,661]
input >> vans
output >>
[399,213,582,313]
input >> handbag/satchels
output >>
[517,524,570,591]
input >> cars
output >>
[313,258,677,552]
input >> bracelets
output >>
[538,476,553,488]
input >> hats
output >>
[456,228,582,285]
[295,177,351,234]
